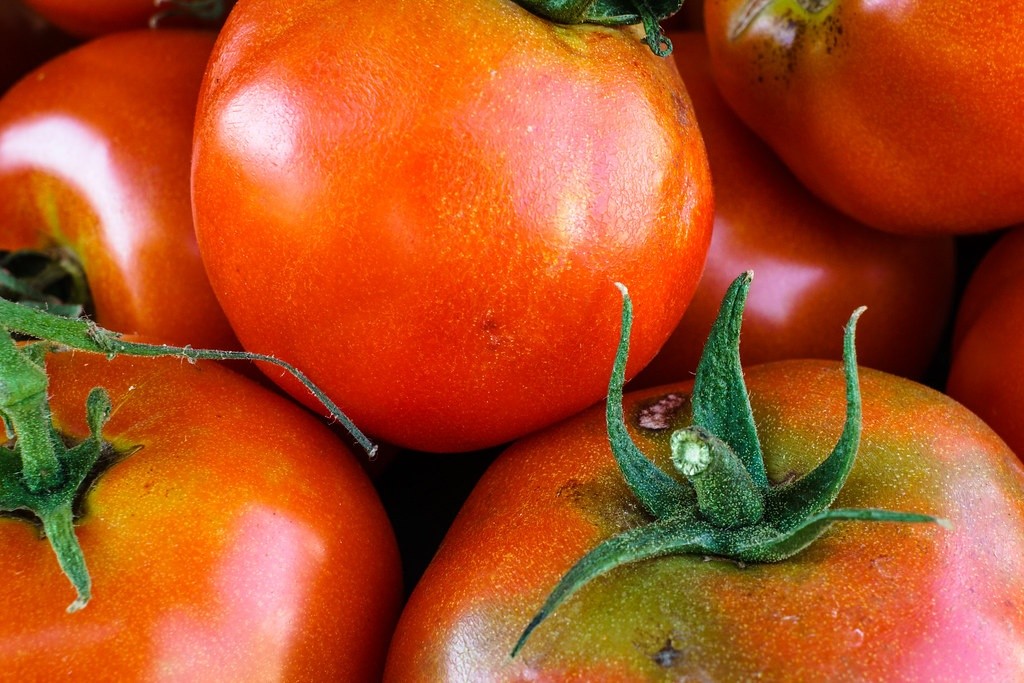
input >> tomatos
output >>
[0,1,1024,683]
